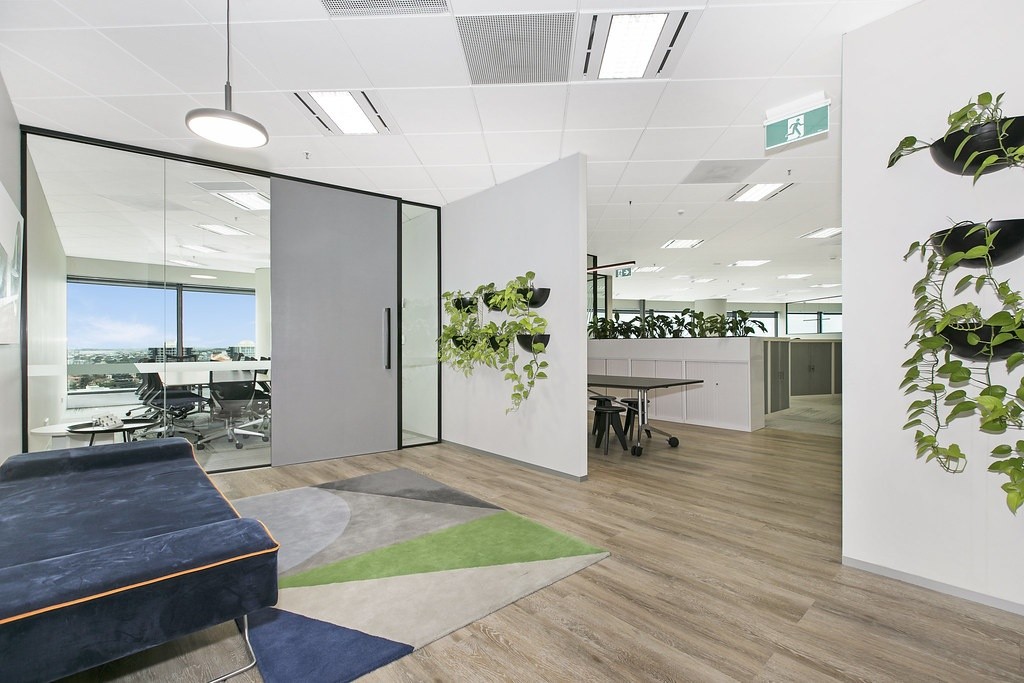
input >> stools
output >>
[589,395,616,435]
[621,398,652,438]
[594,406,628,456]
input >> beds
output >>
[0,434,281,683]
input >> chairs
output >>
[124,350,271,451]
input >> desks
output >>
[587,373,705,457]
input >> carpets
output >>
[223,467,611,683]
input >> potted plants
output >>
[451,319,478,351]
[513,271,550,308]
[930,219,1024,261]
[442,290,478,314]
[514,312,550,354]
[483,282,509,311]
[479,321,514,352]
[927,303,1024,359]
[930,91,1024,177]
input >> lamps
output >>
[185,0,272,148]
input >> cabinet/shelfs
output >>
[586,337,842,434]
